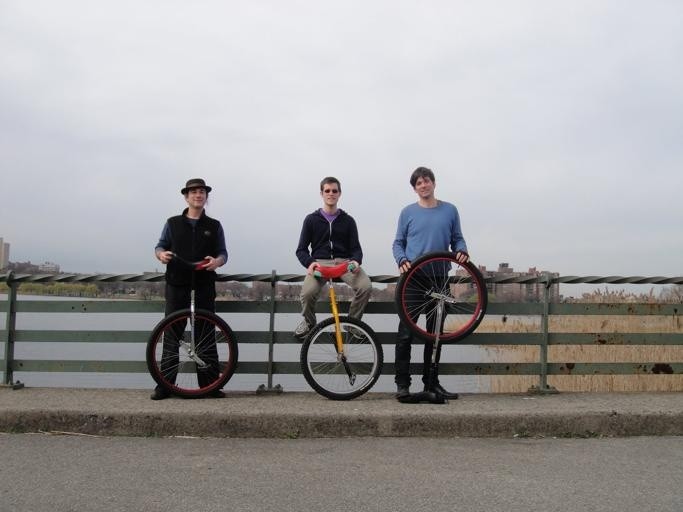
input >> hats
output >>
[180,178,212,195]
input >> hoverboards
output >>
[391,247,495,407]
[143,248,247,402]
[298,261,386,402]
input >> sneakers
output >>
[150,384,174,400]
[343,325,368,340]
[292,319,315,339]
[207,390,227,399]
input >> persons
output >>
[150,178,228,400]
[392,167,471,399]
[294,178,372,341]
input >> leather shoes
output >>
[423,383,458,400]
[396,383,410,399]
